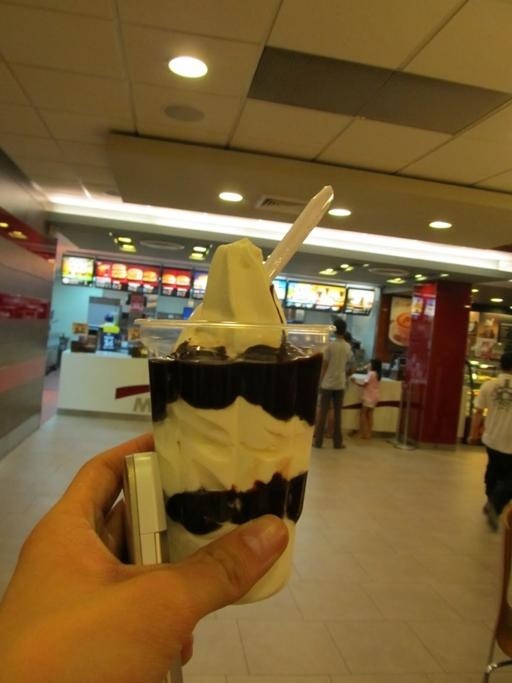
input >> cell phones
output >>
[122,450,183,682]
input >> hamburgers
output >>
[110,263,190,287]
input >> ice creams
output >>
[148,182,336,604]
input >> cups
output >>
[132,317,336,605]
[398,313,410,343]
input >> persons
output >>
[100,314,121,351]
[311,318,355,449]
[467,352,512,533]
[1,430,291,683]
[349,358,383,440]
[314,331,352,439]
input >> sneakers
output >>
[482,501,500,532]
